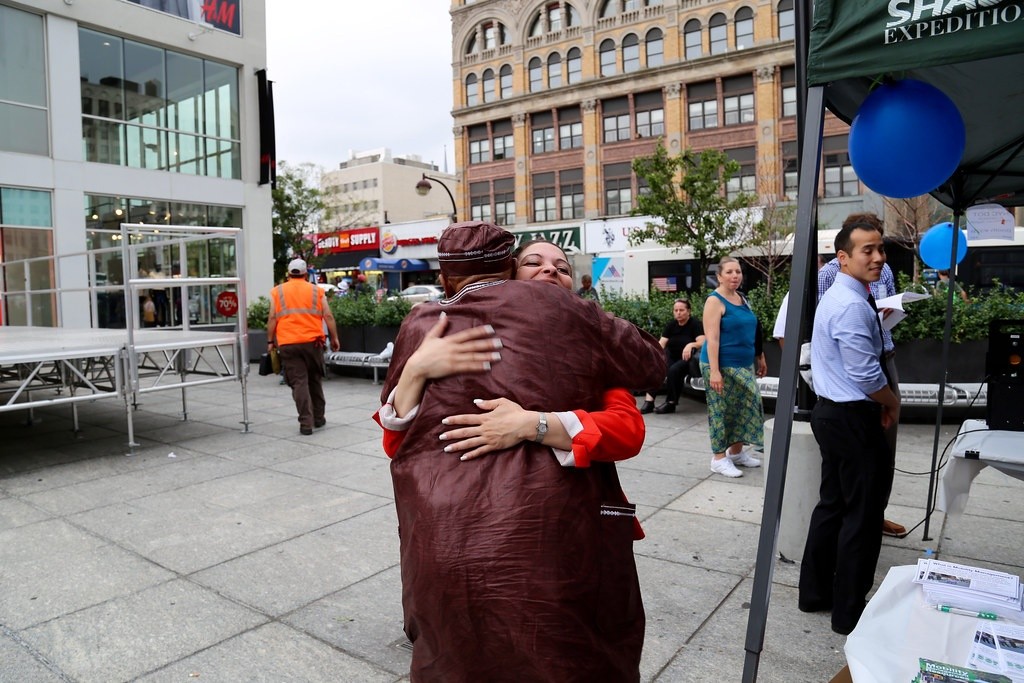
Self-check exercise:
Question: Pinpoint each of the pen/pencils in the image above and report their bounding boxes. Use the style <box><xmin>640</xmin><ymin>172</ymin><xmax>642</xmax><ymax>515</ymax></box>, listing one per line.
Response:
<box><xmin>932</xmin><ymin>603</ymin><xmax>1005</xmax><ymax>621</ymax></box>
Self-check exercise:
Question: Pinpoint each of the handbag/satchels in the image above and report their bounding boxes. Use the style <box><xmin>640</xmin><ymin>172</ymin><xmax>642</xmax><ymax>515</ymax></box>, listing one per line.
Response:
<box><xmin>271</xmin><ymin>351</ymin><xmax>280</xmax><ymax>374</ymax></box>
<box><xmin>258</xmin><ymin>353</ymin><xmax>273</xmax><ymax>375</ymax></box>
<box><xmin>738</xmin><ymin>292</ymin><xmax>763</xmax><ymax>357</ymax></box>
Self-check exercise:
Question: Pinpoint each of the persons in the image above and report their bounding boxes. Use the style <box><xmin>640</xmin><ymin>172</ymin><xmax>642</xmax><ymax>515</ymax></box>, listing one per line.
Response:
<box><xmin>818</xmin><ymin>212</ymin><xmax>909</xmax><ymax>538</ymax></box>
<box><xmin>377</xmin><ymin>274</ymin><xmax>384</xmax><ymax>288</ymax></box>
<box><xmin>138</xmin><ymin>261</ymin><xmax>148</xmax><ymax>328</ymax></box>
<box><xmin>771</xmin><ymin>254</ymin><xmax>825</xmax><ymax>412</ymax></box>
<box><xmin>937</xmin><ymin>269</ymin><xmax>968</xmax><ymax>300</ymax></box>
<box><xmin>698</xmin><ymin>255</ymin><xmax>766</xmax><ymax>478</ymax></box>
<box><xmin>379</xmin><ymin>219</ymin><xmax>671</xmax><ymax>683</ymax></box>
<box><xmin>637</xmin><ymin>298</ymin><xmax>705</xmax><ymax>414</ymax></box>
<box><xmin>797</xmin><ymin>223</ymin><xmax>904</xmax><ymax>634</ymax></box>
<box><xmin>370</xmin><ymin>240</ymin><xmax>645</xmax><ymax>543</ymax></box>
<box><xmin>354</xmin><ymin>274</ymin><xmax>375</xmax><ymax>301</ymax></box>
<box><xmin>266</xmin><ymin>259</ymin><xmax>340</xmax><ymax>435</ymax></box>
<box><xmin>576</xmin><ymin>274</ymin><xmax>600</xmax><ymax>301</ymax></box>
<box><xmin>148</xmin><ymin>263</ymin><xmax>171</xmax><ymax>328</ymax></box>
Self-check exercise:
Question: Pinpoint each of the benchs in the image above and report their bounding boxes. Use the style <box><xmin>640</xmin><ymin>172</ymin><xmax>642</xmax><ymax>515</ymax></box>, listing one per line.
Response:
<box><xmin>323</xmin><ymin>352</ymin><xmax>392</xmax><ymax>386</ymax></box>
<box><xmin>664</xmin><ymin>376</ymin><xmax>989</xmax><ymax>424</ymax></box>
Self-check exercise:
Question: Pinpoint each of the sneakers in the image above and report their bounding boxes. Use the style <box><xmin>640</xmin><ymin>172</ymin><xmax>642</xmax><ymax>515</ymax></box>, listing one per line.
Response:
<box><xmin>711</xmin><ymin>456</ymin><xmax>743</xmax><ymax>477</ymax></box>
<box><xmin>728</xmin><ymin>452</ymin><xmax>761</xmax><ymax>467</ymax></box>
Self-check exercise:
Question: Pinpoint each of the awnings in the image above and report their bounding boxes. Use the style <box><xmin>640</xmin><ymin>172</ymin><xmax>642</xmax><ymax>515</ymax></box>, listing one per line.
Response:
<box><xmin>358</xmin><ymin>257</ymin><xmax>396</xmax><ymax>292</ymax></box>
<box><xmin>394</xmin><ymin>258</ymin><xmax>428</xmax><ymax>292</ymax></box>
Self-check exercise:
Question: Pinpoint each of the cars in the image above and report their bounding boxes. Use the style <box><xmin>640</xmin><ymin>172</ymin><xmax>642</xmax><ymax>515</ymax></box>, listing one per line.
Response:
<box><xmin>387</xmin><ymin>285</ymin><xmax>446</xmax><ymax>304</ymax></box>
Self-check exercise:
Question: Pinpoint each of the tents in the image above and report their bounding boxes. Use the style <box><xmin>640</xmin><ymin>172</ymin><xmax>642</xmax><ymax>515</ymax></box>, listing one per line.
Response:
<box><xmin>741</xmin><ymin>2</ymin><xmax>1024</xmax><ymax>683</ymax></box>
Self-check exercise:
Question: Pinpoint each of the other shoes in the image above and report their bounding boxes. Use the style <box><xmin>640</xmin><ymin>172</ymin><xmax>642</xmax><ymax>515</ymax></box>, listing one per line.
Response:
<box><xmin>882</xmin><ymin>519</ymin><xmax>906</xmax><ymax>537</ymax></box>
<box><xmin>639</xmin><ymin>400</ymin><xmax>656</xmax><ymax>414</ymax></box>
<box><xmin>300</xmin><ymin>428</ymin><xmax>312</xmax><ymax>435</ymax></box>
<box><xmin>315</xmin><ymin>417</ymin><xmax>326</xmax><ymax>427</ymax></box>
<box><xmin>832</xmin><ymin>608</ymin><xmax>863</xmax><ymax>635</ymax></box>
<box><xmin>798</xmin><ymin>596</ymin><xmax>831</xmax><ymax>611</ymax></box>
<box><xmin>653</xmin><ymin>402</ymin><xmax>676</xmax><ymax>413</ymax></box>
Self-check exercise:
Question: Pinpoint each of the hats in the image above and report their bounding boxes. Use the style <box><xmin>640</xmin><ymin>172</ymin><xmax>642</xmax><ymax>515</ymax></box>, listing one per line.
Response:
<box><xmin>288</xmin><ymin>259</ymin><xmax>307</xmax><ymax>275</ymax></box>
<box><xmin>437</xmin><ymin>221</ymin><xmax>516</xmax><ymax>276</ymax></box>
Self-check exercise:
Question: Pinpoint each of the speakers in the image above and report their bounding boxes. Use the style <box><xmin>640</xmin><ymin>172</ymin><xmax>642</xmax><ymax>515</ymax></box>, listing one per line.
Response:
<box><xmin>986</xmin><ymin>319</ymin><xmax>1024</xmax><ymax>431</ymax></box>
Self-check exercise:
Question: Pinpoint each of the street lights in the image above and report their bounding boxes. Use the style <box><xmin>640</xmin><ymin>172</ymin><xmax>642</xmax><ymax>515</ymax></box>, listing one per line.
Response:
<box><xmin>414</xmin><ymin>172</ymin><xmax>457</xmax><ymax>224</ymax></box>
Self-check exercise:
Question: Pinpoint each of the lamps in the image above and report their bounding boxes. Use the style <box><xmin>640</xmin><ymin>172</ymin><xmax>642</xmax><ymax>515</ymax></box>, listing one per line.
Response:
<box><xmin>188</xmin><ymin>21</ymin><xmax>213</xmax><ymax>42</ymax></box>
<box><xmin>416</xmin><ymin>173</ymin><xmax>460</xmax><ymax>214</ymax></box>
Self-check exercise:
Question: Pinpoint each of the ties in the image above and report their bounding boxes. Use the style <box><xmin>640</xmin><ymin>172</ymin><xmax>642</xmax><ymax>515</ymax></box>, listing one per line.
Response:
<box><xmin>868</xmin><ymin>295</ymin><xmax>893</xmax><ymax>390</ymax></box>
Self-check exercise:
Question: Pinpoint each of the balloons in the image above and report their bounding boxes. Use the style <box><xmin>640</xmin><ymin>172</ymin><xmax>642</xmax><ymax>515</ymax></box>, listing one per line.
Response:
<box><xmin>918</xmin><ymin>222</ymin><xmax>968</xmax><ymax>271</ymax></box>
<box><xmin>848</xmin><ymin>79</ymin><xmax>965</xmax><ymax>199</ymax></box>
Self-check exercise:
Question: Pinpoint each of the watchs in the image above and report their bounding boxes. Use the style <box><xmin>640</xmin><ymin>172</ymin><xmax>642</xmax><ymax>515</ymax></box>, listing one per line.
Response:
<box><xmin>536</xmin><ymin>410</ymin><xmax>550</xmax><ymax>444</ymax></box>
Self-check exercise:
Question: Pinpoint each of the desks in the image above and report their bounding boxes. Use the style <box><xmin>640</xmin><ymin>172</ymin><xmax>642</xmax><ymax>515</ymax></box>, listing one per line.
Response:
<box><xmin>829</xmin><ymin>564</ymin><xmax>1024</xmax><ymax>683</ymax></box>
<box><xmin>934</xmin><ymin>419</ymin><xmax>1024</xmax><ymax>561</ymax></box>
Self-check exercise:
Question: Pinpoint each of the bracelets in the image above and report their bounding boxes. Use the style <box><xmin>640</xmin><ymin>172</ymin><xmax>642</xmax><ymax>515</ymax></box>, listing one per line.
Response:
<box><xmin>267</xmin><ymin>341</ymin><xmax>274</xmax><ymax>344</ymax></box>
